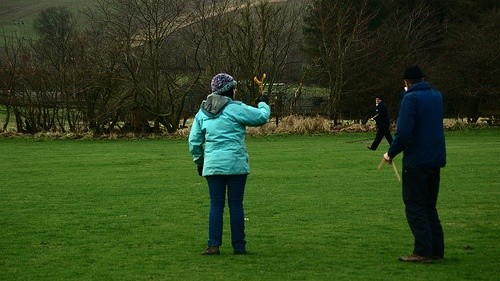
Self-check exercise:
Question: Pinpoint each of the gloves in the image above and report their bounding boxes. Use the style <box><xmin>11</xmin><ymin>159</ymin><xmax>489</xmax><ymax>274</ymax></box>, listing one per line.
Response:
<box><xmin>193</xmin><ymin>156</ymin><xmax>203</xmax><ymax>176</ymax></box>
<box><xmin>258</xmin><ymin>95</ymin><xmax>268</xmax><ymax>104</ymax></box>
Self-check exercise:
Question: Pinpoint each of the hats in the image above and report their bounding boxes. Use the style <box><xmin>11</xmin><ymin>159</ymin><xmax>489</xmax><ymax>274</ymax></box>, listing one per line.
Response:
<box><xmin>210</xmin><ymin>73</ymin><xmax>238</xmax><ymax>94</ymax></box>
<box><xmin>375</xmin><ymin>95</ymin><xmax>384</xmax><ymax>101</ymax></box>
<box><xmin>399</xmin><ymin>66</ymin><xmax>425</xmax><ymax>79</ymax></box>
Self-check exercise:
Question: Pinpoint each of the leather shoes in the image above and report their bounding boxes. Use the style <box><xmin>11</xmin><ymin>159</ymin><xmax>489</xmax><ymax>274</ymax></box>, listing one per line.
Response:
<box><xmin>398</xmin><ymin>254</ymin><xmax>437</xmax><ymax>262</ymax></box>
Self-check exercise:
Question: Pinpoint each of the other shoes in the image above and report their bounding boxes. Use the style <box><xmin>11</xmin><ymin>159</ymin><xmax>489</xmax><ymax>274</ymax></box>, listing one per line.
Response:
<box><xmin>201</xmin><ymin>246</ymin><xmax>221</xmax><ymax>255</ymax></box>
<box><xmin>389</xmin><ymin>143</ymin><xmax>394</xmax><ymax>150</ymax></box>
<box><xmin>367</xmin><ymin>146</ymin><xmax>375</xmax><ymax>150</ymax></box>
<box><xmin>233</xmin><ymin>248</ymin><xmax>248</xmax><ymax>254</ymax></box>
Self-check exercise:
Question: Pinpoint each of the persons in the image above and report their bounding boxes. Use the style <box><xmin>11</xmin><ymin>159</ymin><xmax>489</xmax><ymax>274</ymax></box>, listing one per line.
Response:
<box><xmin>188</xmin><ymin>74</ymin><xmax>270</xmax><ymax>255</ymax></box>
<box><xmin>367</xmin><ymin>96</ymin><xmax>393</xmax><ymax>151</ymax></box>
<box><xmin>384</xmin><ymin>66</ymin><xmax>447</xmax><ymax>263</ymax></box>
